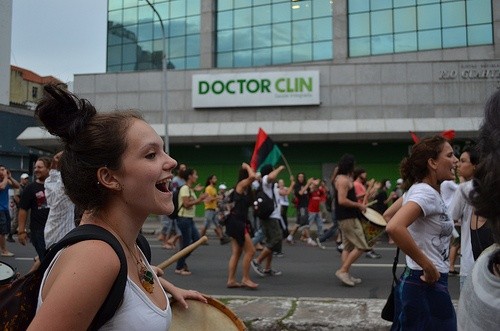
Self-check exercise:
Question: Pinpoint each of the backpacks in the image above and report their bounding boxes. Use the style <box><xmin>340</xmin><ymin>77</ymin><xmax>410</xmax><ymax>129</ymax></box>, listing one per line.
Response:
<box><xmin>0</xmin><ymin>225</ymin><xmax>127</xmax><ymax>331</ymax></box>
<box><xmin>252</xmin><ymin>180</ymin><xmax>278</xmax><ymax>220</ymax></box>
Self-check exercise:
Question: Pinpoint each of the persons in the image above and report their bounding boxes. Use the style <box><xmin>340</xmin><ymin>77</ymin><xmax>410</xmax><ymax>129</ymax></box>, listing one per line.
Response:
<box><xmin>0</xmin><ymin>82</ymin><xmax>500</xmax><ymax>331</ymax></box>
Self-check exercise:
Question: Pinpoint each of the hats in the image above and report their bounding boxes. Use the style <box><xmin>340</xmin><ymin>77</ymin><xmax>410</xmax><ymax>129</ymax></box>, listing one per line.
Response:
<box><xmin>261</xmin><ymin>164</ymin><xmax>273</xmax><ymax>171</ymax></box>
<box><xmin>21</xmin><ymin>174</ymin><xmax>29</xmax><ymax>179</ymax></box>
<box><xmin>219</xmin><ymin>184</ymin><xmax>227</xmax><ymax>190</ymax></box>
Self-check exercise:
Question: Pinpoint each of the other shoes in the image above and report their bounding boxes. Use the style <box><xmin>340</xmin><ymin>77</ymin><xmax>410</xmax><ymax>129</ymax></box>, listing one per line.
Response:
<box><xmin>220</xmin><ymin>235</ymin><xmax>231</xmax><ymax>244</ymax></box>
<box><xmin>242</xmin><ymin>279</ymin><xmax>258</xmax><ymax>288</ymax></box>
<box><xmin>227</xmin><ymin>282</ymin><xmax>242</xmax><ymax>287</ymax></box>
<box><xmin>263</xmin><ymin>269</ymin><xmax>282</xmax><ymax>275</ymax></box>
<box><xmin>7</xmin><ymin>237</ymin><xmax>16</xmax><ymax>243</ymax></box>
<box><xmin>251</xmin><ymin>260</ymin><xmax>264</xmax><ymax>276</ymax></box>
<box><xmin>273</xmin><ymin>252</ymin><xmax>285</xmax><ymax>257</ymax></box>
<box><xmin>350</xmin><ymin>275</ymin><xmax>362</xmax><ymax>284</ymax></box>
<box><xmin>336</xmin><ymin>269</ymin><xmax>355</xmax><ymax>286</ymax></box>
<box><xmin>160</xmin><ymin>234</ymin><xmax>166</xmax><ymax>239</ymax></box>
<box><xmin>448</xmin><ymin>271</ymin><xmax>460</xmax><ymax>276</ymax></box>
<box><xmin>175</xmin><ymin>268</ymin><xmax>191</xmax><ymax>274</ymax></box>
<box><xmin>162</xmin><ymin>243</ymin><xmax>175</xmax><ymax>248</ymax></box>
<box><xmin>287</xmin><ymin>235</ymin><xmax>380</xmax><ymax>259</ymax></box>
<box><xmin>1</xmin><ymin>251</ymin><xmax>14</xmax><ymax>256</ymax></box>
<box><xmin>255</xmin><ymin>242</ymin><xmax>264</xmax><ymax>250</ymax></box>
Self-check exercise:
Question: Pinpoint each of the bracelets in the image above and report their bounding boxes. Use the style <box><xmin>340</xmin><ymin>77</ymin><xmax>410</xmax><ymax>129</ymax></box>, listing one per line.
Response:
<box><xmin>18</xmin><ymin>231</ymin><xmax>26</xmax><ymax>235</ymax></box>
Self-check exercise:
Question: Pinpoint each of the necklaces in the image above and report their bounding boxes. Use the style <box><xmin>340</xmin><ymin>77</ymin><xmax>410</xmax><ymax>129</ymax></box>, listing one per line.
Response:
<box><xmin>108</xmin><ymin>225</ymin><xmax>155</xmax><ymax>294</ymax></box>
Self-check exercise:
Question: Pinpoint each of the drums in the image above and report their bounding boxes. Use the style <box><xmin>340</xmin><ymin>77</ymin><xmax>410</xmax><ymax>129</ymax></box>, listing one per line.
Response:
<box><xmin>342</xmin><ymin>207</ymin><xmax>388</xmax><ymax>253</ymax></box>
<box><xmin>0</xmin><ymin>260</ymin><xmax>18</xmax><ymax>296</ymax></box>
<box><xmin>166</xmin><ymin>294</ymin><xmax>249</xmax><ymax>331</ymax></box>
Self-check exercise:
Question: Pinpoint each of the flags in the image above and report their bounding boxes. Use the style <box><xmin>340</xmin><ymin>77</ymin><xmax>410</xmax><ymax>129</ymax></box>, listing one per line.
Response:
<box><xmin>250</xmin><ymin>128</ymin><xmax>281</xmax><ymax>174</ymax></box>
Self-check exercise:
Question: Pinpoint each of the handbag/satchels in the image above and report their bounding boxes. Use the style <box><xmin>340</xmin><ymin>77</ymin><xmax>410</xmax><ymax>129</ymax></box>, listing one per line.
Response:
<box><xmin>381</xmin><ymin>288</ymin><xmax>395</xmax><ymax>322</ymax></box>
<box><xmin>169</xmin><ymin>209</ymin><xmax>178</xmax><ymax>219</ymax></box>
